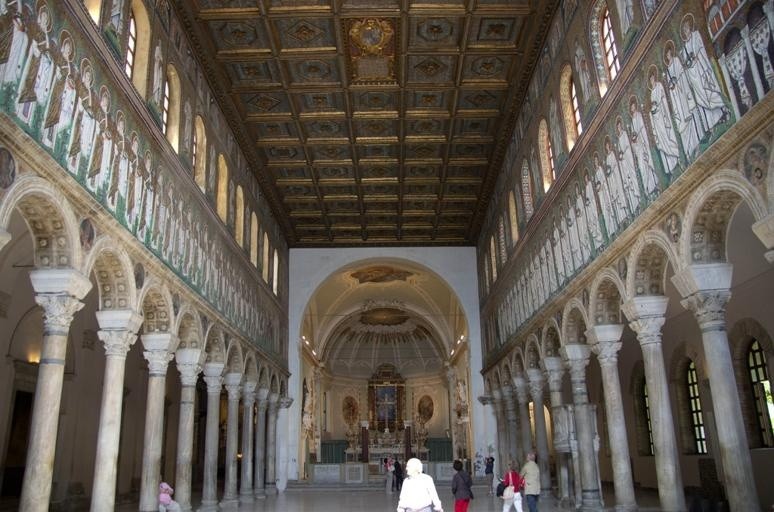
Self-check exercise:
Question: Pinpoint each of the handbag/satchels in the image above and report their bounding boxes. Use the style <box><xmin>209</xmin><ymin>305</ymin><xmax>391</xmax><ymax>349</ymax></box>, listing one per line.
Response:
<box><xmin>499</xmin><ymin>472</ymin><xmax>515</xmax><ymax>500</ymax></box>
<box><xmin>468</xmin><ymin>489</ymin><xmax>476</xmax><ymax>500</ymax></box>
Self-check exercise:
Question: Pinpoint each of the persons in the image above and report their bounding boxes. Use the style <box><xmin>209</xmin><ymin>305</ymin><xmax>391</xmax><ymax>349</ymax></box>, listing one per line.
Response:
<box><xmin>159</xmin><ymin>474</ymin><xmax>180</xmax><ymax>512</ymax></box>
<box><xmin>384</xmin><ymin>453</ymin><xmax>541</xmax><ymax>512</ymax></box>
